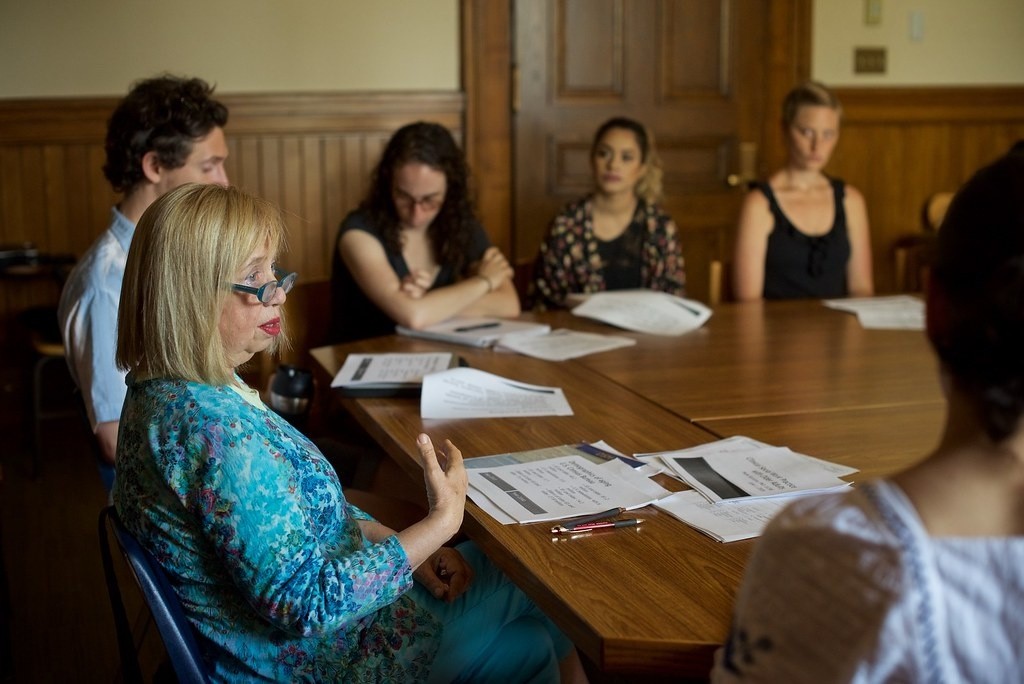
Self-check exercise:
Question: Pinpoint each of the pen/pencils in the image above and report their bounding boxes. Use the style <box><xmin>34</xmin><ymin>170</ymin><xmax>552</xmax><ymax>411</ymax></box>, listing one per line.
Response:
<box><xmin>548</xmin><ymin>506</ymin><xmax>647</xmax><ymax>534</ymax></box>
<box><xmin>455</xmin><ymin>322</ymin><xmax>501</xmax><ymax>332</ymax></box>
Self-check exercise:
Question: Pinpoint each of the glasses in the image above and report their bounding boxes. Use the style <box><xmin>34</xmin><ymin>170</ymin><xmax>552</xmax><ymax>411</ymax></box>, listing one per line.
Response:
<box><xmin>220</xmin><ymin>262</ymin><xmax>298</xmax><ymax>303</ymax></box>
<box><xmin>389</xmin><ymin>183</ymin><xmax>445</xmax><ymax>211</ymax></box>
<box><xmin>790</xmin><ymin>122</ymin><xmax>834</xmax><ymax>143</ymax></box>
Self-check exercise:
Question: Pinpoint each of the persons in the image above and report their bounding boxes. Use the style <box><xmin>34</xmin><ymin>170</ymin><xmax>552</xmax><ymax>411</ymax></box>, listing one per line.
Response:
<box><xmin>114</xmin><ymin>183</ymin><xmax>589</xmax><ymax>684</ymax></box>
<box><xmin>59</xmin><ymin>78</ymin><xmax>468</xmax><ymax>547</ymax></box>
<box><xmin>711</xmin><ymin>142</ymin><xmax>1023</xmax><ymax>684</ymax></box>
<box><xmin>732</xmin><ymin>82</ymin><xmax>874</xmax><ymax>300</ymax></box>
<box><xmin>329</xmin><ymin>121</ymin><xmax>521</xmax><ymax>344</ymax></box>
<box><xmin>533</xmin><ymin>116</ymin><xmax>687</xmax><ymax>311</ymax></box>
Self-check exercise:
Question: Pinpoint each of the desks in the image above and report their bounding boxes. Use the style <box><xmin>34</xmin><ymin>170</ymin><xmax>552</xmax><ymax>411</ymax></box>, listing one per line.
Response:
<box><xmin>309</xmin><ymin>294</ymin><xmax>948</xmax><ymax>681</ymax></box>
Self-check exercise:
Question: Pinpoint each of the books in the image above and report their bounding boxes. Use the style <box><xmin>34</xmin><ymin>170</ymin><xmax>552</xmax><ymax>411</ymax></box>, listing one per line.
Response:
<box><xmin>395</xmin><ymin>317</ymin><xmax>552</xmax><ymax>347</ymax></box>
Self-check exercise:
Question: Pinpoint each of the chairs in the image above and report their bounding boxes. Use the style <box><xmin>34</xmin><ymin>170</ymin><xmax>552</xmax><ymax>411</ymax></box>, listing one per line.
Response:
<box><xmin>99</xmin><ymin>510</ymin><xmax>209</xmax><ymax>684</ymax></box>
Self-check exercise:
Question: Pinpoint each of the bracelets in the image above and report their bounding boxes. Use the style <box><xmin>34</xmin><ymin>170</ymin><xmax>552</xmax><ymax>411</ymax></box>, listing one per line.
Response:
<box><xmin>473</xmin><ymin>273</ymin><xmax>492</xmax><ymax>292</ymax></box>
